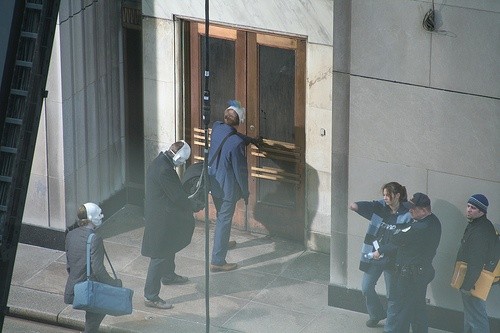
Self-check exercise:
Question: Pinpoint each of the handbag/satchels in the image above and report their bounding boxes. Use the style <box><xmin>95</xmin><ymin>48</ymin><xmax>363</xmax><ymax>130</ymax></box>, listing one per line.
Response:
<box><xmin>181</xmin><ymin>160</ymin><xmax>209</xmax><ymax>211</ymax></box>
<box><xmin>73</xmin><ymin>281</ymin><xmax>133</xmax><ymax>315</ymax></box>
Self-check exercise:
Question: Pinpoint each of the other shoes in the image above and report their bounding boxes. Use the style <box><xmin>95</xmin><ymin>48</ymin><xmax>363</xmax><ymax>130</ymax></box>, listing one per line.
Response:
<box><xmin>228</xmin><ymin>241</ymin><xmax>235</xmax><ymax>248</ymax></box>
<box><xmin>367</xmin><ymin>314</ymin><xmax>386</xmax><ymax>326</ymax></box>
<box><xmin>210</xmin><ymin>263</ymin><xmax>236</xmax><ymax>272</ymax></box>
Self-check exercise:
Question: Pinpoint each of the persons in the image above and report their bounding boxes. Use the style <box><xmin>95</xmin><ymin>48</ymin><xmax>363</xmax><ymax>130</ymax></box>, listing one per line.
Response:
<box><xmin>140</xmin><ymin>139</ymin><xmax>193</xmax><ymax>309</ymax></box>
<box><xmin>65</xmin><ymin>202</ymin><xmax>122</xmax><ymax>333</ymax></box>
<box><xmin>454</xmin><ymin>194</ymin><xmax>500</xmax><ymax>333</ymax></box>
<box><xmin>350</xmin><ymin>182</ymin><xmax>416</xmax><ymax>328</ymax></box>
<box><xmin>373</xmin><ymin>192</ymin><xmax>441</xmax><ymax>333</ymax></box>
<box><xmin>208</xmin><ymin>106</ymin><xmax>264</xmax><ymax>273</ymax></box>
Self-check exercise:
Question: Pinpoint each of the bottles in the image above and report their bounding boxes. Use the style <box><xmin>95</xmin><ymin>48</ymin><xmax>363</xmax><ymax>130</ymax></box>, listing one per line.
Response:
<box><xmin>364</xmin><ymin>252</ymin><xmax>384</xmax><ymax>259</ymax></box>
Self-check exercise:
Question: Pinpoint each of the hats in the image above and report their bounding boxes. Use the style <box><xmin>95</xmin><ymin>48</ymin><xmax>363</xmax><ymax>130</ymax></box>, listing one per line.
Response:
<box><xmin>467</xmin><ymin>194</ymin><xmax>489</xmax><ymax>214</ymax></box>
<box><xmin>402</xmin><ymin>192</ymin><xmax>430</xmax><ymax>209</ymax></box>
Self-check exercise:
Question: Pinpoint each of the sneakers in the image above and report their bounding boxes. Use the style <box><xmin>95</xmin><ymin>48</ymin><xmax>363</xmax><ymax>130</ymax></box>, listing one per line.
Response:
<box><xmin>145</xmin><ymin>297</ymin><xmax>172</xmax><ymax>309</ymax></box>
<box><xmin>162</xmin><ymin>275</ymin><xmax>189</xmax><ymax>285</ymax></box>
